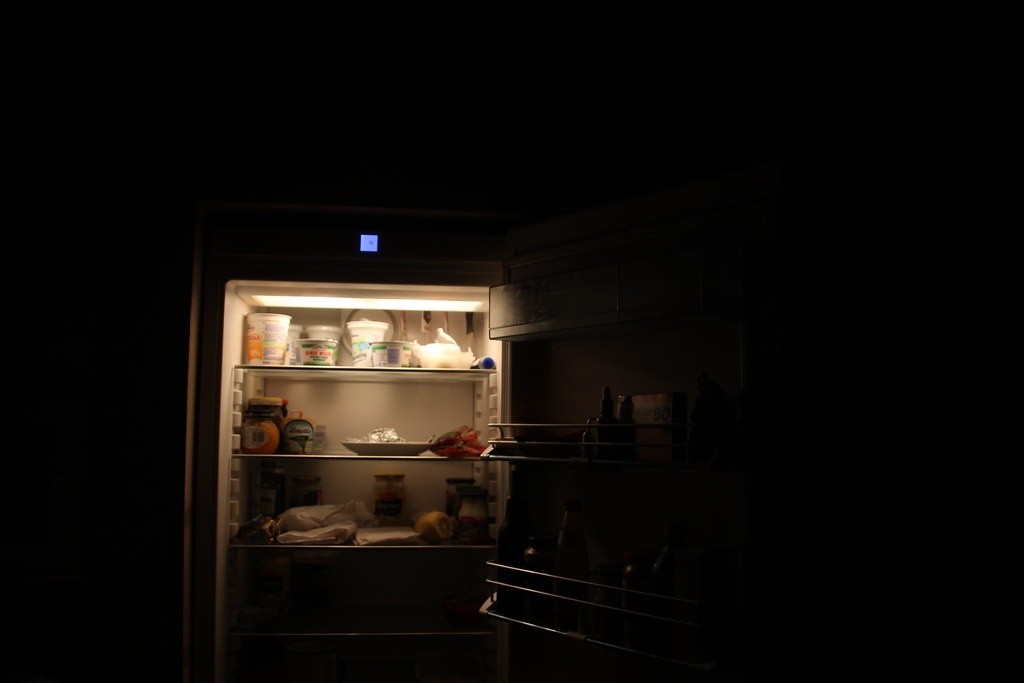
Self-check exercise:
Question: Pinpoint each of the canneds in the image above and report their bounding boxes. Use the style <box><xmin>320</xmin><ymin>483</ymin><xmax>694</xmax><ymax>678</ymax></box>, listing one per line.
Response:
<box><xmin>445</xmin><ymin>478</ymin><xmax>490</xmax><ymax>543</ymax></box>
<box><xmin>253</xmin><ymin>468</ymin><xmax>286</xmax><ymax>516</ymax></box>
<box><xmin>295</xmin><ymin>476</ymin><xmax>321</xmax><ymax>506</ymax></box>
<box><xmin>374</xmin><ymin>474</ymin><xmax>405</xmax><ymax>523</ymax></box>
<box><xmin>241</xmin><ymin>412</ymin><xmax>279</xmax><ymax>454</ymax></box>
<box><xmin>250</xmin><ymin>397</ymin><xmax>283</xmax><ymax>451</ymax></box>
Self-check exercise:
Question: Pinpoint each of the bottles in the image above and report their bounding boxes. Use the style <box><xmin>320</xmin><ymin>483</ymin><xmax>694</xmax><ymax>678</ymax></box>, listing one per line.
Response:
<box><xmin>595</xmin><ymin>376</ymin><xmax>637</xmax><ymax>458</ymax></box>
<box><xmin>497</xmin><ymin>461</ymin><xmax>688</xmax><ymax>657</ymax></box>
<box><xmin>445</xmin><ymin>474</ymin><xmax>490</xmax><ymax>544</ymax></box>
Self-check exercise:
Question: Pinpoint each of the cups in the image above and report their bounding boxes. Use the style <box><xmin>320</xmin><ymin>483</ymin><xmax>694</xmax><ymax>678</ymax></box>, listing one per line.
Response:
<box><xmin>246</xmin><ymin>311</ymin><xmax>414</xmax><ymax>369</ymax></box>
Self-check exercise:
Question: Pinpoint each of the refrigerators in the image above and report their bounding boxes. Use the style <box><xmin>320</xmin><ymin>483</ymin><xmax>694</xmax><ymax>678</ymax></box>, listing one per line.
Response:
<box><xmin>175</xmin><ymin>163</ymin><xmax>769</xmax><ymax>683</ymax></box>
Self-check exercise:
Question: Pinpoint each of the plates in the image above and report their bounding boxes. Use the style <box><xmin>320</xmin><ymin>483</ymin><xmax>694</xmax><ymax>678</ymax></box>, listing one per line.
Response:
<box><xmin>338</xmin><ymin>438</ymin><xmax>436</xmax><ymax>455</ymax></box>
